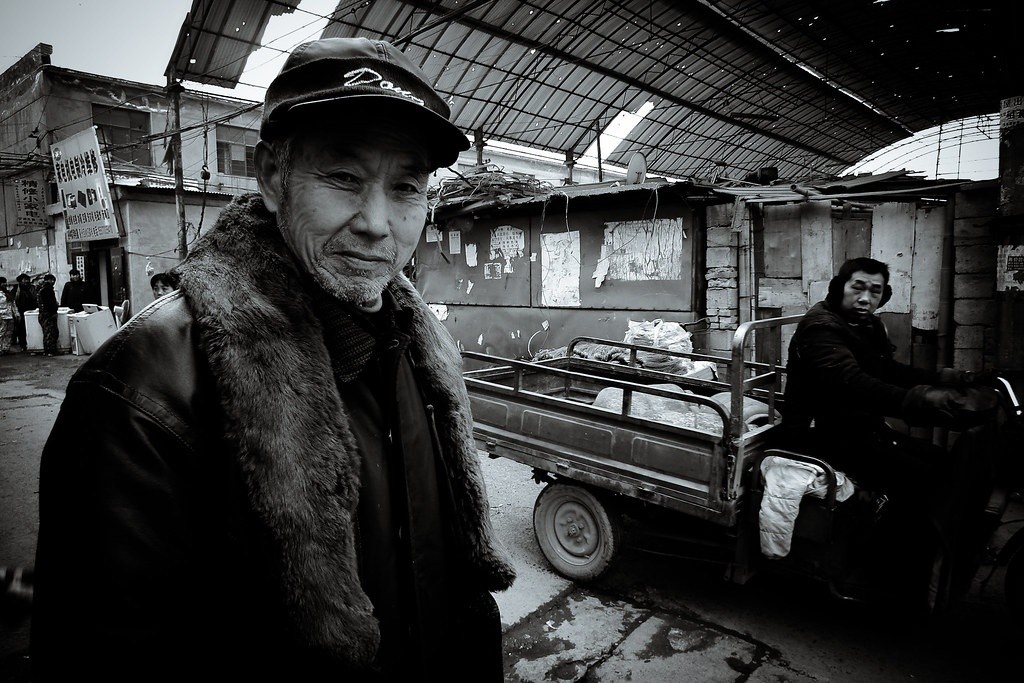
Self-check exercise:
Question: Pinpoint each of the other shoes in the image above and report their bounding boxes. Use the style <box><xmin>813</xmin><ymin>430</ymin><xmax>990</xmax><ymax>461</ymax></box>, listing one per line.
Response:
<box><xmin>44</xmin><ymin>351</ymin><xmax>64</xmax><ymax>357</ymax></box>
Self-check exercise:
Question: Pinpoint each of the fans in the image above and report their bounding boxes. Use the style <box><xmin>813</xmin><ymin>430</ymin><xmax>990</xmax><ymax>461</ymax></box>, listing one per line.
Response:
<box><xmin>113</xmin><ymin>300</ymin><xmax>129</xmax><ymax>330</ymax></box>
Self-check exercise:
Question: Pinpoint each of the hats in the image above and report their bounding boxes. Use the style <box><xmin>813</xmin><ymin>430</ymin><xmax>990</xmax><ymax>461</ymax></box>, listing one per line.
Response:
<box><xmin>260</xmin><ymin>35</ymin><xmax>469</xmax><ymax>166</ymax></box>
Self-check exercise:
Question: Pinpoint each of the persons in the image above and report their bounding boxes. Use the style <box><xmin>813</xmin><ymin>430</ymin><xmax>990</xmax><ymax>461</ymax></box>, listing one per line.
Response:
<box><xmin>27</xmin><ymin>37</ymin><xmax>513</xmax><ymax>683</ymax></box>
<box><xmin>150</xmin><ymin>273</ymin><xmax>175</xmax><ymax>299</ymax></box>
<box><xmin>60</xmin><ymin>269</ymin><xmax>89</xmax><ymax>313</ymax></box>
<box><xmin>0</xmin><ymin>274</ymin><xmax>36</xmax><ymax>355</ymax></box>
<box><xmin>781</xmin><ymin>258</ymin><xmax>978</xmax><ymax>606</ymax></box>
<box><xmin>36</xmin><ymin>274</ymin><xmax>65</xmax><ymax>356</ymax></box>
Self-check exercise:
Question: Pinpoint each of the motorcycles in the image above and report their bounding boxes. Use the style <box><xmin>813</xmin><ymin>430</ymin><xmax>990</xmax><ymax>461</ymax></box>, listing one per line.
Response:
<box><xmin>458</xmin><ymin>313</ymin><xmax>1023</xmax><ymax>617</ymax></box>
